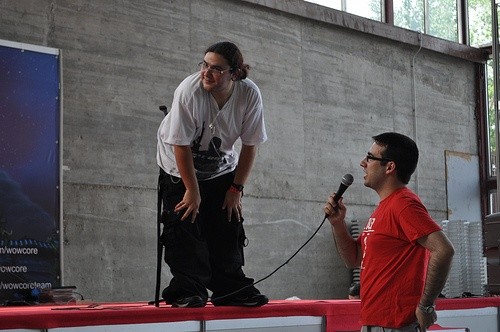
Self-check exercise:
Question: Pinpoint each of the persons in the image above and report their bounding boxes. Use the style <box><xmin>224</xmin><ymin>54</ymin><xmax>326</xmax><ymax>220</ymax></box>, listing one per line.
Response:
<box><xmin>324</xmin><ymin>132</ymin><xmax>455</xmax><ymax>332</ymax></box>
<box><xmin>156</xmin><ymin>41</ymin><xmax>268</xmax><ymax>308</ymax></box>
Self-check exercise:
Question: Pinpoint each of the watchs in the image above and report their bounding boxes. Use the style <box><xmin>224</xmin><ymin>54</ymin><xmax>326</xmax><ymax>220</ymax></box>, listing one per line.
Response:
<box><xmin>419</xmin><ymin>304</ymin><xmax>435</xmax><ymax>313</ymax></box>
<box><xmin>229</xmin><ymin>183</ymin><xmax>244</xmax><ymax>192</ymax></box>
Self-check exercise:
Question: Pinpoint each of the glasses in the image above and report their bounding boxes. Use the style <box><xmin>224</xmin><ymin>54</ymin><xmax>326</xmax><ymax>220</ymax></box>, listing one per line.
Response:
<box><xmin>198</xmin><ymin>62</ymin><xmax>233</xmax><ymax>74</ymax></box>
<box><xmin>366</xmin><ymin>153</ymin><xmax>384</xmax><ymax>162</ymax></box>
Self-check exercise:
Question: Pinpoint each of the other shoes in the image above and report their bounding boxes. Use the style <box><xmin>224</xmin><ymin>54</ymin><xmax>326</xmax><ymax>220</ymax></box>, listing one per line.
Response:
<box><xmin>172</xmin><ymin>297</ymin><xmax>206</xmax><ymax>308</ymax></box>
<box><xmin>212</xmin><ymin>294</ymin><xmax>268</xmax><ymax>307</ymax></box>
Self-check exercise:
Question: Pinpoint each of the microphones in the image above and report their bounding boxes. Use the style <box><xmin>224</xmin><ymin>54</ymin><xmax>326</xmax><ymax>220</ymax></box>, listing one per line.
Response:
<box><xmin>325</xmin><ymin>174</ymin><xmax>354</xmax><ymax>217</ymax></box>
